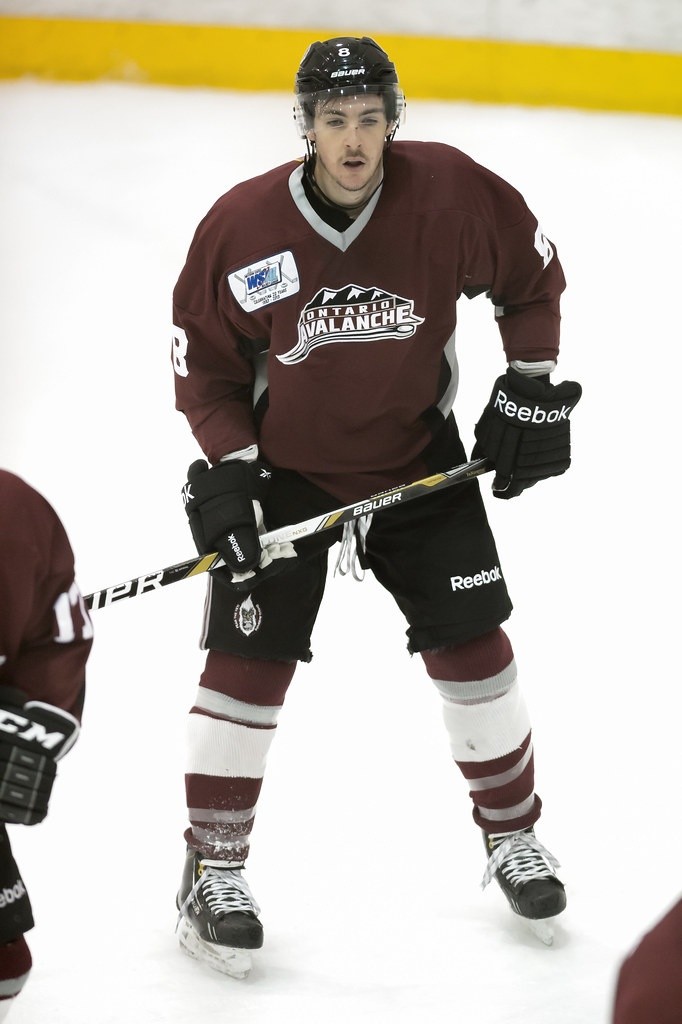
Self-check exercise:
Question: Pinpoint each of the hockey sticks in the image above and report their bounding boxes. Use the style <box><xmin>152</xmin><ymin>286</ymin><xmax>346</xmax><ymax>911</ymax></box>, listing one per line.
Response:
<box><xmin>77</xmin><ymin>456</ymin><xmax>491</xmax><ymax>609</ymax></box>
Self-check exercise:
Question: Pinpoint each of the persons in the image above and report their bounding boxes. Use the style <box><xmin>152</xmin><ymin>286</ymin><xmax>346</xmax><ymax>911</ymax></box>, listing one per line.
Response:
<box><xmin>0</xmin><ymin>466</ymin><xmax>95</xmax><ymax>1024</ymax></box>
<box><xmin>171</xmin><ymin>32</ymin><xmax>582</xmax><ymax>979</ymax></box>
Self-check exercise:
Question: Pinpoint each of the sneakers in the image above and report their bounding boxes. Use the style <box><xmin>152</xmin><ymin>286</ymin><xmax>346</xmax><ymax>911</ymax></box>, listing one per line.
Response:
<box><xmin>176</xmin><ymin>828</ymin><xmax>263</xmax><ymax>979</ymax></box>
<box><xmin>472</xmin><ymin>794</ymin><xmax>566</xmax><ymax>946</ymax></box>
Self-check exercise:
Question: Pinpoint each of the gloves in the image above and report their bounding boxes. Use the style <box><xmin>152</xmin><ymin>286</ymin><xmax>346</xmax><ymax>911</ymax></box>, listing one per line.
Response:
<box><xmin>0</xmin><ymin>700</ymin><xmax>81</xmax><ymax>826</ymax></box>
<box><xmin>180</xmin><ymin>460</ymin><xmax>298</xmax><ymax>592</ymax></box>
<box><xmin>470</xmin><ymin>367</ymin><xmax>582</xmax><ymax>500</ymax></box>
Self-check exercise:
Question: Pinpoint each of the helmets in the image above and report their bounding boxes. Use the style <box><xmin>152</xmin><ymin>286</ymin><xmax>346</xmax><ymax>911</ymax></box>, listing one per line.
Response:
<box><xmin>296</xmin><ymin>36</ymin><xmax>399</xmax><ymax>125</ymax></box>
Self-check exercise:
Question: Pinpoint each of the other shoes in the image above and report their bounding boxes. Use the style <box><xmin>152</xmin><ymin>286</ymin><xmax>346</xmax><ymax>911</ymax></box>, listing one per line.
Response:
<box><xmin>0</xmin><ymin>935</ymin><xmax>32</xmax><ymax>1000</ymax></box>
